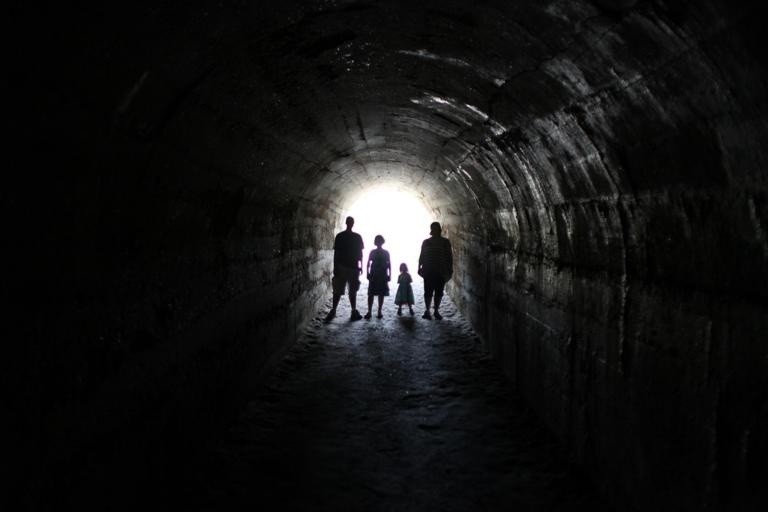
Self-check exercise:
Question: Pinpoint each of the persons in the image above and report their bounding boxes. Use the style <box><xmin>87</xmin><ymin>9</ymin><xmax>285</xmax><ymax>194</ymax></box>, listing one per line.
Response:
<box><xmin>417</xmin><ymin>222</ymin><xmax>453</xmax><ymax>320</ymax></box>
<box><xmin>365</xmin><ymin>235</ymin><xmax>391</xmax><ymax>319</ymax></box>
<box><xmin>394</xmin><ymin>263</ymin><xmax>415</xmax><ymax>315</ymax></box>
<box><xmin>324</xmin><ymin>216</ymin><xmax>364</xmax><ymax>322</ymax></box>
